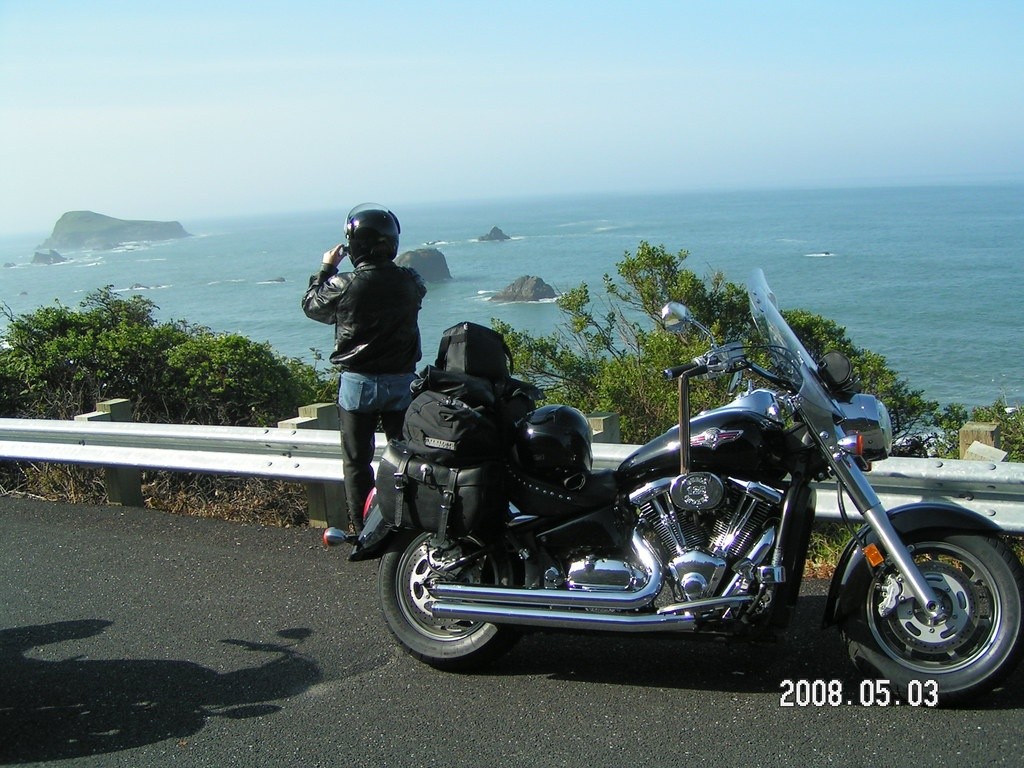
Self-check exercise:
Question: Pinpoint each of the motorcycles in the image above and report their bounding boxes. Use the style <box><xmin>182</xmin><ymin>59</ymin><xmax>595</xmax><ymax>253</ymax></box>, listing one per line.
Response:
<box><xmin>321</xmin><ymin>267</ymin><xmax>1023</xmax><ymax>709</ymax></box>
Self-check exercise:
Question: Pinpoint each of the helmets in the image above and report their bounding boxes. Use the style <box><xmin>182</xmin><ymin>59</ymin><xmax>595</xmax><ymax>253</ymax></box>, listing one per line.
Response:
<box><xmin>513</xmin><ymin>402</ymin><xmax>593</xmax><ymax>484</ymax></box>
<box><xmin>344</xmin><ymin>203</ymin><xmax>400</xmax><ymax>267</ymax></box>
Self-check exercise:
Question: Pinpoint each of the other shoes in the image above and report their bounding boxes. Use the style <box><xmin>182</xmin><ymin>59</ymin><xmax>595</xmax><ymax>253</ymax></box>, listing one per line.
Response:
<box><xmin>342</xmin><ymin>521</ymin><xmax>362</xmax><ymax>545</ymax></box>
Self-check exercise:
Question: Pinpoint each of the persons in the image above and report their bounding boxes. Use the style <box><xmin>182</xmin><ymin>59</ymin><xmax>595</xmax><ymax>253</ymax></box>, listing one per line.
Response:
<box><xmin>302</xmin><ymin>201</ymin><xmax>427</xmax><ymax>542</ymax></box>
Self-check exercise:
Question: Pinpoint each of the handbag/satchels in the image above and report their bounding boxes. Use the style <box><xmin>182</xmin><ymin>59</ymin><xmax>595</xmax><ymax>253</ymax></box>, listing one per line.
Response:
<box><xmin>434</xmin><ymin>321</ymin><xmax>515</xmax><ymax>380</ymax></box>
<box><xmin>375</xmin><ymin>439</ymin><xmax>487</xmax><ymax>545</ymax></box>
<box><xmin>401</xmin><ymin>389</ymin><xmax>506</xmax><ymax>469</ymax></box>
